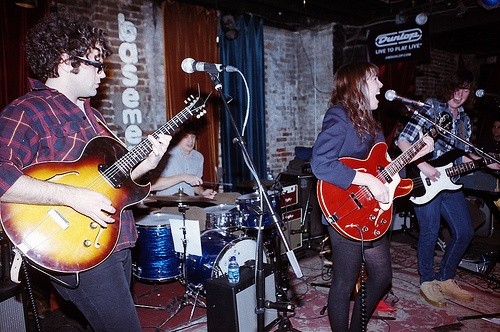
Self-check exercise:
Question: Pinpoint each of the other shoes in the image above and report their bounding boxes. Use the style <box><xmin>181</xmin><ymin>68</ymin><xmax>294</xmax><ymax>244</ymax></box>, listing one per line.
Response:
<box><xmin>419</xmin><ymin>280</ymin><xmax>448</xmax><ymax>308</ymax></box>
<box><xmin>433</xmin><ymin>278</ymin><xmax>475</xmax><ymax>303</ymax></box>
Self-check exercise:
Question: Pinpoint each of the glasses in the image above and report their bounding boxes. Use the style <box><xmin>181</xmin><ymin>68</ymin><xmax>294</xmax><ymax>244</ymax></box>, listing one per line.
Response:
<box><xmin>74</xmin><ymin>55</ymin><xmax>106</xmax><ymax>74</ymax></box>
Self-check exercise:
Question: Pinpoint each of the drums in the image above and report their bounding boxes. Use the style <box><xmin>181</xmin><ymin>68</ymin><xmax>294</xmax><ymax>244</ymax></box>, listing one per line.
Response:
<box><xmin>179</xmin><ymin>230</ymin><xmax>268</xmax><ymax>295</ymax></box>
<box><xmin>132</xmin><ymin>214</ymin><xmax>186</xmax><ymax>281</ymax></box>
<box><xmin>237</xmin><ymin>190</ymin><xmax>280</xmax><ymax>229</ymax></box>
<box><xmin>203</xmin><ymin>205</ymin><xmax>242</xmax><ymax>230</ymax></box>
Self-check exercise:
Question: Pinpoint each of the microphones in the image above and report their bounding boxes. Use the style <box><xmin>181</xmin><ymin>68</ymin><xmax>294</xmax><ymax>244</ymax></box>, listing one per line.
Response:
<box><xmin>385</xmin><ymin>89</ymin><xmax>434</xmax><ymax>109</ymax></box>
<box><xmin>290</xmin><ymin>229</ymin><xmax>310</xmax><ymax>235</ymax></box>
<box><xmin>476</xmin><ymin>89</ymin><xmax>500</xmax><ymax>98</ymax></box>
<box><xmin>181</xmin><ymin>58</ymin><xmax>238</xmax><ymax>73</ymax></box>
<box><xmin>264</xmin><ymin>300</ymin><xmax>295</xmax><ymax>311</ymax></box>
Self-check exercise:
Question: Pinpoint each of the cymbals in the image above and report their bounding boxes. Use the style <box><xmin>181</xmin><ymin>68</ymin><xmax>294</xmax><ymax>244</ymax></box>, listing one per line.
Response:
<box><xmin>146</xmin><ymin>193</ymin><xmax>217</xmax><ymax>202</ymax></box>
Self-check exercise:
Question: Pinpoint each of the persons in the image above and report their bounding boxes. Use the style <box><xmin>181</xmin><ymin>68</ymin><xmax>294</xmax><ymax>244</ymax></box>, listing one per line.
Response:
<box><xmin>147</xmin><ymin>124</ymin><xmax>217</xmax><ymax>200</ymax></box>
<box><xmin>309</xmin><ymin>61</ymin><xmax>434</xmax><ymax>332</ymax></box>
<box><xmin>394</xmin><ymin>68</ymin><xmax>500</xmax><ymax>308</ymax></box>
<box><xmin>0</xmin><ymin>9</ymin><xmax>172</xmax><ymax>332</ymax></box>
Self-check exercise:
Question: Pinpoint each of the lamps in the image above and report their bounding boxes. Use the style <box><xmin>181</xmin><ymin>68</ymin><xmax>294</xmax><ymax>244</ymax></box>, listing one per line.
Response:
<box><xmin>221</xmin><ymin>14</ymin><xmax>238</xmax><ymax>41</ymax></box>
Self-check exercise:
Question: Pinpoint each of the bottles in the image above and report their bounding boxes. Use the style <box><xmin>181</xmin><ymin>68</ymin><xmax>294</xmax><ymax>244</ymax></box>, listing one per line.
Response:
<box><xmin>228</xmin><ymin>256</ymin><xmax>240</xmax><ymax>284</ymax></box>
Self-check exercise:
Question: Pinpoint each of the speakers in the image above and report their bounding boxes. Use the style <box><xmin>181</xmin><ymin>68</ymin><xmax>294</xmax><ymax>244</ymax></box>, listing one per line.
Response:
<box><xmin>207</xmin><ymin>260</ymin><xmax>281</xmax><ymax>332</ymax></box>
<box><xmin>276</xmin><ymin>170</ymin><xmax>328</xmax><ymax>256</ymax></box>
<box><xmin>0</xmin><ymin>280</ymin><xmax>27</xmax><ymax>332</ymax></box>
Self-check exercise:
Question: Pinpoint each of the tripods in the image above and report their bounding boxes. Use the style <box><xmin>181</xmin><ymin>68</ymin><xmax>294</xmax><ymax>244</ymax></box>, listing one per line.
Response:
<box><xmin>157</xmin><ymin>204</ymin><xmax>207</xmax><ymax>332</ymax></box>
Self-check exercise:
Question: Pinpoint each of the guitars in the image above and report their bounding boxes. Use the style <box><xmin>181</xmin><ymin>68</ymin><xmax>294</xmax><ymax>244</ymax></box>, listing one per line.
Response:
<box><xmin>316</xmin><ymin>111</ymin><xmax>453</xmax><ymax>241</ymax></box>
<box><xmin>0</xmin><ymin>94</ymin><xmax>208</xmax><ymax>274</ymax></box>
<box><xmin>407</xmin><ymin>149</ymin><xmax>500</xmax><ymax>205</ymax></box>
